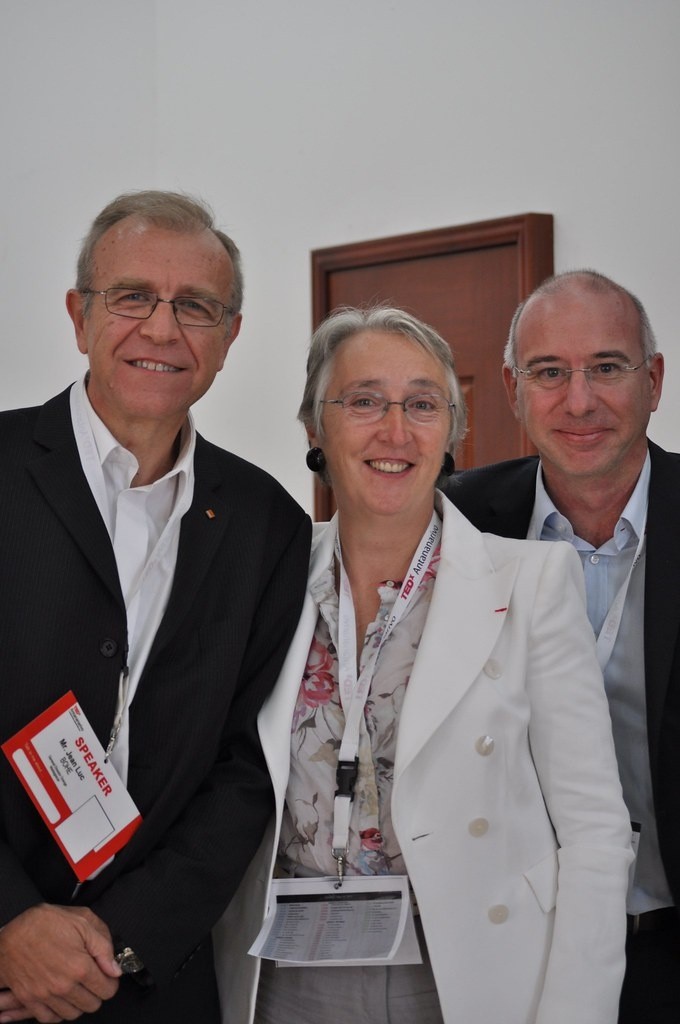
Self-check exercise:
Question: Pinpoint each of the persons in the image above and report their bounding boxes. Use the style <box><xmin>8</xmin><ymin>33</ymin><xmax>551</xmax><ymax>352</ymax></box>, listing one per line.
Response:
<box><xmin>210</xmin><ymin>308</ymin><xmax>632</xmax><ymax>1023</ymax></box>
<box><xmin>0</xmin><ymin>189</ymin><xmax>315</xmax><ymax>1023</ymax></box>
<box><xmin>435</xmin><ymin>269</ymin><xmax>680</xmax><ymax>1023</ymax></box>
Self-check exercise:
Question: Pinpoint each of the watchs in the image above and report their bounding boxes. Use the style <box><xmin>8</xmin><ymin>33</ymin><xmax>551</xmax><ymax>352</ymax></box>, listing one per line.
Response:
<box><xmin>112</xmin><ymin>937</ymin><xmax>145</xmax><ymax>974</ymax></box>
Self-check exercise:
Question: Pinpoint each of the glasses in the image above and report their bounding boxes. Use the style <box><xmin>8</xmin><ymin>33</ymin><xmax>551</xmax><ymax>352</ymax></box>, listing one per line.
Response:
<box><xmin>515</xmin><ymin>357</ymin><xmax>652</xmax><ymax>390</ymax></box>
<box><xmin>80</xmin><ymin>287</ymin><xmax>236</xmax><ymax>327</ymax></box>
<box><xmin>318</xmin><ymin>392</ymin><xmax>455</xmax><ymax>426</ymax></box>
